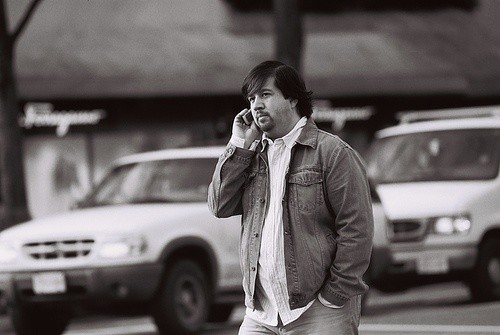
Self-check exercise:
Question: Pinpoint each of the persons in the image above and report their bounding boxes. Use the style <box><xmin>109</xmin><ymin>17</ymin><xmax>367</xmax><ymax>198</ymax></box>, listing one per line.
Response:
<box><xmin>206</xmin><ymin>58</ymin><xmax>375</xmax><ymax>335</ymax></box>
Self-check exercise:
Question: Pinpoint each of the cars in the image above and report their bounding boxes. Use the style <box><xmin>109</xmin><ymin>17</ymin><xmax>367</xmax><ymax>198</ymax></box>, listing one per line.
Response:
<box><xmin>0</xmin><ymin>141</ymin><xmax>396</xmax><ymax>334</ymax></box>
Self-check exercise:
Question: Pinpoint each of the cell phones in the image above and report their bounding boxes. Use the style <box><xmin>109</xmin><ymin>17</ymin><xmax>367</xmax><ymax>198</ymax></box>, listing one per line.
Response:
<box><xmin>242</xmin><ymin>108</ymin><xmax>254</xmax><ymax>125</ymax></box>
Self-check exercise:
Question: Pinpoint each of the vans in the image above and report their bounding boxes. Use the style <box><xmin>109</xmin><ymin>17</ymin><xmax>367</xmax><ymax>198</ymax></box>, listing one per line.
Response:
<box><xmin>364</xmin><ymin>116</ymin><xmax>499</xmax><ymax>301</ymax></box>
